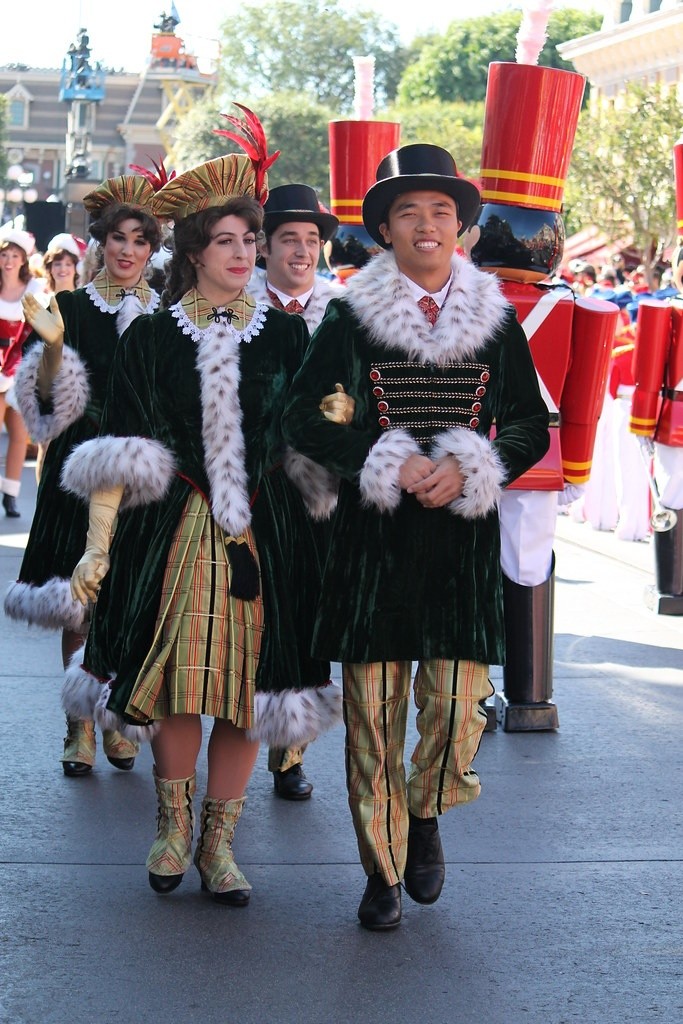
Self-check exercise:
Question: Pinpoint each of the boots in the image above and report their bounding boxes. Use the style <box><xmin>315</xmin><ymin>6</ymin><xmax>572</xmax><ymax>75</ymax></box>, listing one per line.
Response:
<box><xmin>0</xmin><ymin>478</ymin><xmax>20</xmax><ymax>517</ymax></box>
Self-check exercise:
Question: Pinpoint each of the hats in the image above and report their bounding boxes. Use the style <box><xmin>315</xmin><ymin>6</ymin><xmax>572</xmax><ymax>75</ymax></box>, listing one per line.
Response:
<box><xmin>47</xmin><ymin>233</ymin><xmax>83</xmax><ymax>276</ymax></box>
<box><xmin>83</xmin><ymin>153</ymin><xmax>172</xmax><ymax>228</ymax></box>
<box><xmin>256</xmin><ymin>184</ymin><xmax>339</xmax><ymax>271</ymax></box>
<box><xmin>151</xmin><ymin>103</ymin><xmax>280</xmax><ymax>222</ymax></box>
<box><xmin>2</xmin><ymin>229</ymin><xmax>36</xmax><ymax>254</ymax></box>
<box><xmin>362</xmin><ymin>144</ymin><xmax>480</xmax><ymax>251</ymax></box>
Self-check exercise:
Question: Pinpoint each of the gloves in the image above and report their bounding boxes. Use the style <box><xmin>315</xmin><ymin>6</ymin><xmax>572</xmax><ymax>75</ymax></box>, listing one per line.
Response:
<box><xmin>70</xmin><ymin>484</ymin><xmax>124</xmax><ymax>608</ymax></box>
<box><xmin>319</xmin><ymin>382</ymin><xmax>355</xmax><ymax>424</ymax></box>
<box><xmin>21</xmin><ymin>293</ymin><xmax>64</xmax><ymax>402</ymax></box>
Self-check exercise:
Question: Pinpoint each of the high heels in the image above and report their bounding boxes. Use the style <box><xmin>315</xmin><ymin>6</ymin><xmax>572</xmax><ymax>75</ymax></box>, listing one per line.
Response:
<box><xmin>148</xmin><ymin>872</ymin><xmax>184</xmax><ymax>893</ymax></box>
<box><xmin>60</xmin><ymin>761</ymin><xmax>91</xmax><ymax>776</ymax></box>
<box><xmin>193</xmin><ymin>854</ymin><xmax>250</xmax><ymax>906</ymax></box>
<box><xmin>108</xmin><ymin>757</ymin><xmax>134</xmax><ymax>770</ymax></box>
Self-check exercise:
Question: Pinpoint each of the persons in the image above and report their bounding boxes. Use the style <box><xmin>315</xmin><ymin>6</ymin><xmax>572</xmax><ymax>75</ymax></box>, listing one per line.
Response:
<box><xmin>0</xmin><ymin>228</ymin><xmax>87</xmax><ymax>519</ymax></box>
<box><xmin>278</xmin><ymin>144</ymin><xmax>552</xmax><ymax>929</ymax></box>
<box><xmin>3</xmin><ymin>155</ymin><xmax>176</xmax><ymax>774</ymax></box>
<box><xmin>470</xmin><ymin>0</ymin><xmax>618</xmax><ymax>731</ymax></box>
<box><xmin>569</xmin><ymin>239</ymin><xmax>683</xmax><ymax>302</ymax></box>
<box><xmin>325</xmin><ymin>59</ymin><xmax>399</xmax><ymax>287</ymax></box>
<box><xmin>63</xmin><ymin>103</ymin><xmax>357</xmax><ymax>904</ymax></box>
<box><xmin>628</xmin><ymin>144</ymin><xmax>683</xmax><ymax>616</ymax></box>
<box><xmin>248</xmin><ymin>185</ymin><xmax>345</xmax><ymax>801</ymax></box>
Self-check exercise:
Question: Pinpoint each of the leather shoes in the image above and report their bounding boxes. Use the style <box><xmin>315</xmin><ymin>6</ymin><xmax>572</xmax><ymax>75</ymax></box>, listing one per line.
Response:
<box><xmin>275</xmin><ymin>765</ymin><xmax>313</xmax><ymax>800</ymax></box>
<box><xmin>406</xmin><ymin>814</ymin><xmax>445</xmax><ymax>905</ymax></box>
<box><xmin>358</xmin><ymin>874</ymin><xmax>401</xmax><ymax>929</ymax></box>
